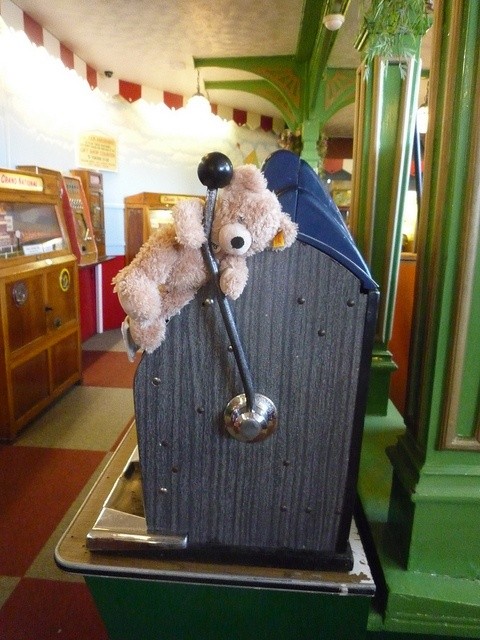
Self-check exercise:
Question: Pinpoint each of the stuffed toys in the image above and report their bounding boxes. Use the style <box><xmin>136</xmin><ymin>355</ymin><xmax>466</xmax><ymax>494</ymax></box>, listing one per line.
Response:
<box><xmin>112</xmin><ymin>163</ymin><xmax>299</xmax><ymax>353</ymax></box>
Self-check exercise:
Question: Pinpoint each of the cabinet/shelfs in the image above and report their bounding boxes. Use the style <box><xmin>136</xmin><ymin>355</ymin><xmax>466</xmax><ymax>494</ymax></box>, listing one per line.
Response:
<box><xmin>0</xmin><ymin>259</ymin><xmax>84</xmax><ymax>441</ymax></box>
